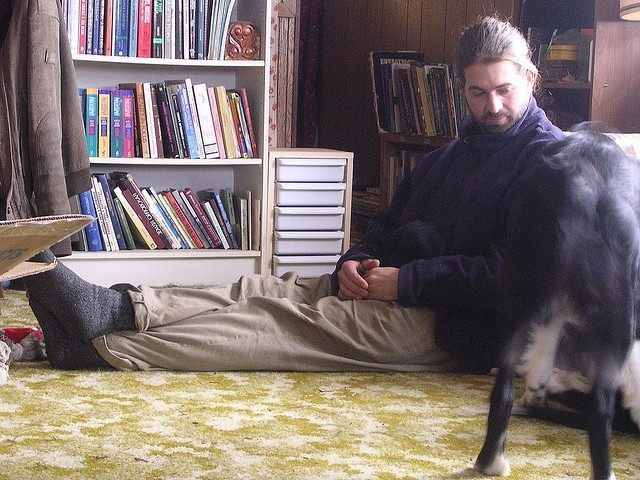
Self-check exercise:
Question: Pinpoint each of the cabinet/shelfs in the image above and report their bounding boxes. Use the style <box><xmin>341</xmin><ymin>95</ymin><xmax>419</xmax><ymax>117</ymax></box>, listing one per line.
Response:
<box><xmin>268</xmin><ymin>147</ymin><xmax>353</xmax><ymax>281</ymax></box>
<box><xmin>523</xmin><ymin>0</ymin><xmax>640</xmax><ymax>132</ymax></box>
<box><xmin>368</xmin><ymin>49</ymin><xmax>458</xmax><ymax>203</ymax></box>
<box><xmin>58</xmin><ymin>0</ymin><xmax>269</xmax><ymax>288</ymax></box>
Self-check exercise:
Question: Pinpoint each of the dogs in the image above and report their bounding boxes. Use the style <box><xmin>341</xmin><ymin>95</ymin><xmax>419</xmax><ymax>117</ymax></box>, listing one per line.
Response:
<box><xmin>472</xmin><ymin>128</ymin><xmax>640</xmax><ymax>480</ymax></box>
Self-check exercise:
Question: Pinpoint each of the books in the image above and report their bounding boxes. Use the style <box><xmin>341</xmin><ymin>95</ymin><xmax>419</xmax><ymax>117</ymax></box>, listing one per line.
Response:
<box><xmin>59</xmin><ymin>0</ymin><xmax>237</xmax><ymax>61</ymax></box>
<box><xmin>352</xmin><ymin>185</ymin><xmax>384</xmax><ymax>225</ymax></box>
<box><xmin>77</xmin><ymin>78</ymin><xmax>259</xmax><ymax>159</ymax></box>
<box><xmin>369</xmin><ymin>48</ymin><xmax>471</xmax><ymax>140</ymax></box>
<box><xmin>68</xmin><ymin>171</ymin><xmax>261</xmax><ymax>250</ymax></box>
<box><xmin>388</xmin><ymin>149</ymin><xmax>423</xmax><ymax>206</ymax></box>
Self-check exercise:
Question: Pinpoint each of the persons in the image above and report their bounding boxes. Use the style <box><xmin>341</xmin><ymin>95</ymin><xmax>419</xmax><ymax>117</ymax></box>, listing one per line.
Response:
<box><xmin>24</xmin><ymin>18</ymin><xmax>568</xmax><ymax>374</ymax></box>
